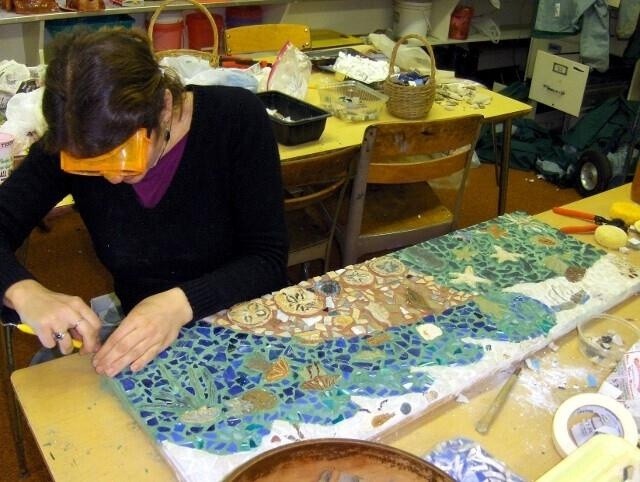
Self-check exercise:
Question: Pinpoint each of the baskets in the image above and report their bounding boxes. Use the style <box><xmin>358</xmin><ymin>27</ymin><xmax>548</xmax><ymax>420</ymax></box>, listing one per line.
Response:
<box><xmin>384</xmin><ymin>33</ymin><xmax>436</xmax><ymax>120</ymax></box>
<box><xmin>146</xmin><ymin>1</ymin><xmax>221</xmax><ymax>69</ymax></box>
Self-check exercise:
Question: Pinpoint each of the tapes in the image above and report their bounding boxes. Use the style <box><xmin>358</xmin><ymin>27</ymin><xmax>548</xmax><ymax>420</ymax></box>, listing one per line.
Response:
<box><xmin>551</xmin><ymin>392</ymin><xmax>638</xmax><ymax>460</ymax></box>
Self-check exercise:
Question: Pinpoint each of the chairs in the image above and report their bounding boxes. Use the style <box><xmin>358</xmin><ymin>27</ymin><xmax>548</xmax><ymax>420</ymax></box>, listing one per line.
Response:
<box><xmin>281</xmin><ymin>113</ymin><xmax>484</xmax><ymax>281</ymax></box>
<box><xmin>224</xmin><ymin>24</ymin><xmax>312</xmax><ymax>57</ymax></box>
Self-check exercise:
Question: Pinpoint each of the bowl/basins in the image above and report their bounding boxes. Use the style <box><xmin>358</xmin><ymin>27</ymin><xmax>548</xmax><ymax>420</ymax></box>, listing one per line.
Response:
<box><xmin>577</xmin><ymin>313</ymin><xmax>640</xmax><ymax>368</ymax></box>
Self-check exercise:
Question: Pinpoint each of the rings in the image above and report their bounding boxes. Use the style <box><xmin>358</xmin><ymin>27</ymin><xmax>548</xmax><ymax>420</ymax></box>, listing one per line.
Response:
<box><xmin>72</xmin><ymin>316</ymin><xmax>84</xmax><ymax>329</ymax></box>
<box><xmin>53</xmin><ymin>332</ymin><xmax>68</xmax><ymax>343</ymax></box>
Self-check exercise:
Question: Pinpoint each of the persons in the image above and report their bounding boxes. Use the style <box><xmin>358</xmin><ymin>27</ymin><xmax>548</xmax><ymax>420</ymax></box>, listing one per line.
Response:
<box><xmin>1</xmin><ymin>29</ymin><xmax>293</xmax><ymax>379</ymax></box>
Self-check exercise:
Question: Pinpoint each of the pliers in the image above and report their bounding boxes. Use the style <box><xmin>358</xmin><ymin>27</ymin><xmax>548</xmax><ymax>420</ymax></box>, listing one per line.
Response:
<box><xmin>553</xmin><ymin>207</ymin><xmax>628</xmax><ymax>234</ymax></box>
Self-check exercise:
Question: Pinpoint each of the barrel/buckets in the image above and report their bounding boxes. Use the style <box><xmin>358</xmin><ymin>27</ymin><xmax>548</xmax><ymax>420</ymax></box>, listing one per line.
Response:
<box><xmin>185</xmin><ymin>11</ymin><xmax>222</xmax><ymax>54</ymax></box>
<box><xmin>225</xmin><ymin>6</ymin><xmax>262</xmax><ymax>28</ymax></box>
<box><xmin>392</xmin><ymin>0</ymin><xmax>433</xmax><ymax>44</ymax></box>
<box><xmin>147</xmin><ymin>10</ymin><xmax>183</xmax><ymax>50</ymax></box>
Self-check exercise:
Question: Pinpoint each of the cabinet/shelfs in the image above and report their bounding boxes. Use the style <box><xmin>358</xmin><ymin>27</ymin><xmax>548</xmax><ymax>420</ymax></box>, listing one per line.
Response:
<box><xmin>0</xmin><ymin>1</ymin><xmax>539</xmax><ymax>48</ymax></box>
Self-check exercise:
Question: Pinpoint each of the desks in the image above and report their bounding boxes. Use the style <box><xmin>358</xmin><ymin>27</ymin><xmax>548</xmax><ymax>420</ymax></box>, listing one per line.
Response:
<box><xmin>0</xmin><ymin>45</ymin><xmax>533</xmax><ymax>217</ymax></box>
<box><xmin>8</xmin><ymin>181</ymin><xmax>639</xmax><ymax>482</ymax></box>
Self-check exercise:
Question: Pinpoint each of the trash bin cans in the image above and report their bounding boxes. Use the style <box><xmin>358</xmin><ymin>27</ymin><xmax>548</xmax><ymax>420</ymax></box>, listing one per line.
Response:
<box><xmin>29</xmin><ymin>292</ymin><xmax>128</xmax><ymax>367</ymax></box>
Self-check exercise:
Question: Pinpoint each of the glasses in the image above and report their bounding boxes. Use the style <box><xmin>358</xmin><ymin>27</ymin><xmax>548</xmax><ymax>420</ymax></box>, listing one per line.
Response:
<box><xmin>59</xmin><ymin>126</ymin><xmax>156</xmax><ymax>180</ymax></box>
